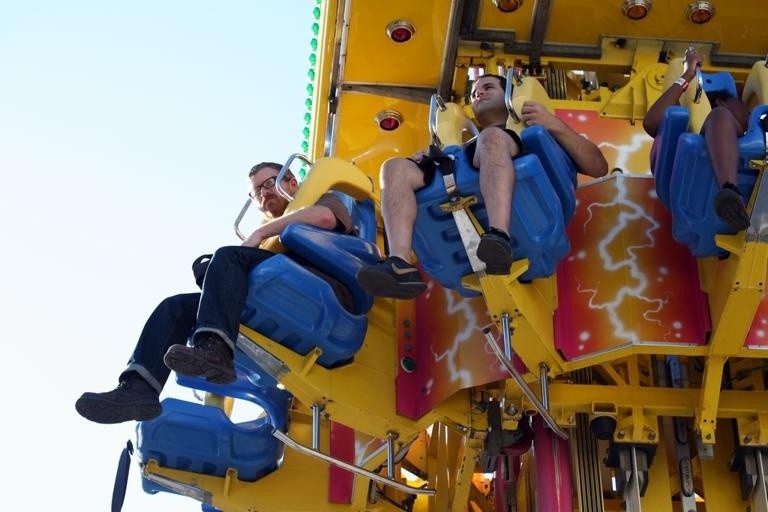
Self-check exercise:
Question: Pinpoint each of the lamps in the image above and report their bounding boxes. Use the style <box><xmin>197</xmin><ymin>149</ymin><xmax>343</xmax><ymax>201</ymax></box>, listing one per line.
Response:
<box><xmin>621</xmin><ymin>0</ymin><xmax>650</xmax><ymax>20</ymax></box>
<box><xmin>489</xmin><ymin>0</ymin><xmax>525</xmax><ymax>12</ymax></box>
<box><xmin>385</xmin><ymin>19</ymin><xmax>416</xmax><ymax>43</ymax></box>
<box><xmin>685</xmin><ymin>1</ymin><xmax>716</xmax><ymax>25</ymax></box>
<box><xmin>372</xmin><ymin>109</ymin><xmax>402</xmax><ymax>131</ymax></box>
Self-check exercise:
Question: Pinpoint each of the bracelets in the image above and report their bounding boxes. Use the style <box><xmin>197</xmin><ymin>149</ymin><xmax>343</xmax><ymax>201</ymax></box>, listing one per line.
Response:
<box><xmin>673</xmin><ymin>78</ymin><xmax>688</xmax><ymax>92</ymax></box>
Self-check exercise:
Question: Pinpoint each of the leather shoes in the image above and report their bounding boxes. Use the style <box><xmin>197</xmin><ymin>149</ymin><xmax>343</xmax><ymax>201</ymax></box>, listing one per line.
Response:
<box><xmin>75</xmin><ymin>375</ymin><xmax>163</xmax><ymax>424</ymax></box>
<box><xmin>164</xmin><ymin>338</ymin><xmax>237</xmax><ymax>385</ymax></box>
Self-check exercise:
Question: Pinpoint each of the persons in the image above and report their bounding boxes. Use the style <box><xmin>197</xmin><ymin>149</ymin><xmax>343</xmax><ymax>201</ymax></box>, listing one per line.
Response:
<box><xmin>357</xmin><ymin>74</ymin><xmax>608</xmax><ymax>301</ymax></box>
<box><xmin>76</xmin><ymin>162</ymin><xmax>358</xmax><ymax>425</ymax></box>
<box><xmin>640</xmin><ymin>48</ymin><xmax>768</xmax><ymax>230</ymax></box>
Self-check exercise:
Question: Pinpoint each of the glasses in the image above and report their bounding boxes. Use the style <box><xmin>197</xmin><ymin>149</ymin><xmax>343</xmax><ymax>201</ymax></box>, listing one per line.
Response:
<box><xmin>248</xmin><ymin>175</ymin><xmax>282</xmax><ymax>199</ymax></box>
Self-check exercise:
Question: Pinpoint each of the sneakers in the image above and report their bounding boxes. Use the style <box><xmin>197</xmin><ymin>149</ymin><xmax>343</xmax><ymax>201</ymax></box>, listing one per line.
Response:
<box><xmin>356</xmin><ymin>257</ymin><xmax>426</xmax><ymax>300</ymax></box>
<box><xmin>476</xmin><ymin>228</ymin><xmax>512</xmax><ymax>276</ymax></box>
<box><xmin>714</xmin><ymin>183</ymin><xmax>751</xmax><ymax>232</ymax></box>
